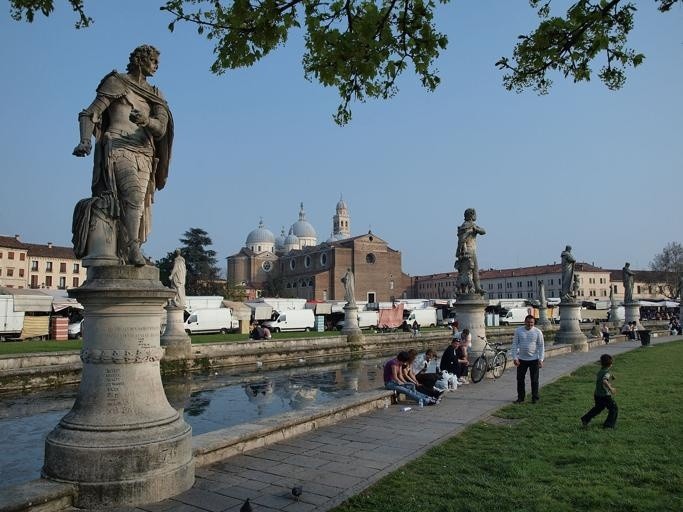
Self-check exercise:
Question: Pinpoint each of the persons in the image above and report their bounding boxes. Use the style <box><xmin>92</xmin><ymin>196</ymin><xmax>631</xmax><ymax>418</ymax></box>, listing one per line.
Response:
<box><xmin>511</xmin><ymin>316</ymin><xmax>545</xmax><ymax>404</ymax></box>
<box><xmin>261</xmin><ymin>325</ymin><xmax>272</xmax><ymax>341</ymax></box>
<box><xmin>561</xmin><ymin>243</ymin><xmax>581</xmax><ymax>300</ymax></box>
<box><xmin>412</xmin><ymin>319</ymin><xmax>422</xmax><ymax>330</ymax></box>
<box><xmin>381</xmin><ymin>330</ymin><xmax>473</xmax><ymax>407</ymax></box>
<box><xmin>537</xmin><ymin>280</ymin><xmax>546</xmax><ymax>302</ymax></box>
<box><xmin>395</xmin><ymin>320</ymin><xmax>412</xmax><ymax>332</ymax></box>
<box><xmin>580</xmin><ymin>354</ymin><xmax>617</xmax><ymax>428</ymax></box>
<box><xmin>167</xmin><ymin>246</ymin><xmax>190</xmax><ymax>309</ymax></box>
<box><xmin>69</xmin><ymin>44</ymin><xmax>176</xmax><ymax>266</ymax></box>
<box><xmin>453</xmin><ymin>208</ymin><xmax>487</xmax><ymax>294</ymax></box>
<box><xmin>247</xmin><ymin>323</ymin><xmax>262</xmax><ymax>339</ymax></box>
<box><xmin>594</xmin><ymin>306</ymin><xmax>683</xmax><ymax>348</ymax></box>
<box><xmin>341</xmin><ymin>265</ymin><xmax>358</xmax><ymax>307</ymax></box>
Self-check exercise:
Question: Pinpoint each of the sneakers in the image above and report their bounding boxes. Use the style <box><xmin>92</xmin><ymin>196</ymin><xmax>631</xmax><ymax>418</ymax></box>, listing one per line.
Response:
<box><xmin>423</xmin><ymin>378</ymin><xmax>469</xmax><ymax>406</ymax></box>
<box><xmin>513</xmin><ymin>400</ymin><xmax>538</xmax><ymax>404</ymax></box>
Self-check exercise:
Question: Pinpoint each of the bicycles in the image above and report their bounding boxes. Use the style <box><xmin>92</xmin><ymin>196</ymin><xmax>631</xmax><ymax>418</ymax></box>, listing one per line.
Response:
<box><xmin>471</xmin><ymin>335</ymin><xmax>508</xmax><ymax>383</ymax></box>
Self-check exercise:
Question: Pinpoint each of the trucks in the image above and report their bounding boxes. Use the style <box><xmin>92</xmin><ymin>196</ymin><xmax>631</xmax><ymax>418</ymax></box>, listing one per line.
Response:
<box><xmin>184</xmin><ymin>308</ymin><xmax>232</xmax><ymax>335</ymax></box>
<box><xmin>261</xmin><ymin>309</ymin><xmax>316</xmax><ymax>333</ymax></box>
<box><xmin>336</xmin><ymin>310</ymin><xmax>379</xmax><ymax>331</ymax></box>
<box><xmin>403</xmin><ymin>308</ymin><xmax>437</xmax><ymax>329</ymax></box>
<box><xmin>498</xmin><ymin>309</ymin><xmax>529</xmax><ymax>325</ymax></box>
